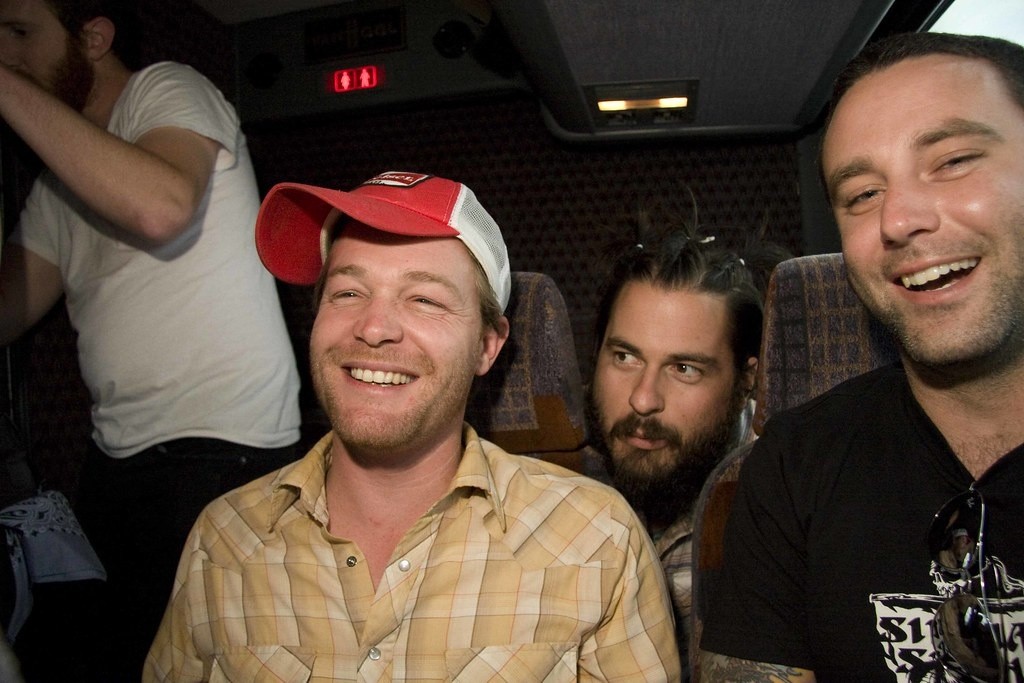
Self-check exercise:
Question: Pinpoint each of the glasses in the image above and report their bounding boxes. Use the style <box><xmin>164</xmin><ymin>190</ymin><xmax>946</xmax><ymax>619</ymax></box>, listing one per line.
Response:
<box><xmin>920</xmin><ymin>481</ymin><xmax>1004</xmax><ymax>683</ymax></box>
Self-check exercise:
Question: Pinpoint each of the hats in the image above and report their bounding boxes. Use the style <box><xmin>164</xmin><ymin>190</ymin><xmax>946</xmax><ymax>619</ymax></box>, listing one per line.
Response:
<box><xmin>254</xmin><ymin>171</ymin><xmax>511</xmax><ymax>320</ymax></box>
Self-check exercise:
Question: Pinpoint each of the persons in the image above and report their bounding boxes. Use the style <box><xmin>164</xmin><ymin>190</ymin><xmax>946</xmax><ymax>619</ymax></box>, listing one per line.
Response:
<box><xmin>0</xmin><ymin>0</ymin><xmax>301</xmax><ymax>683</ymax></box>
<box><xmin>586</xmin><ymin>237</ymin><xmax>764</xmax><ymax>683</ymax></box>
<box><xmin>140</xmin><ymin>170</ymin><xmax>682</xmax><ymax>683</ymax></box>
<box><xmin>698</xmin><ymin>31</ymin><xmax>1024</xmax><ymax>683</ymax></box>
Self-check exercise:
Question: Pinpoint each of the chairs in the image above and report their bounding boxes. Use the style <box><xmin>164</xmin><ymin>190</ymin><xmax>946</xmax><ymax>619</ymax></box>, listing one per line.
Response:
<box><xmin>690</xmin><ymin>252</ymin><xmax>901</xmax><ymax>683</ymax></box>
<box><xmin>461</xmin><ymin>271</ymin><xmax>647</xmax><ymax>529</ymax></box>
